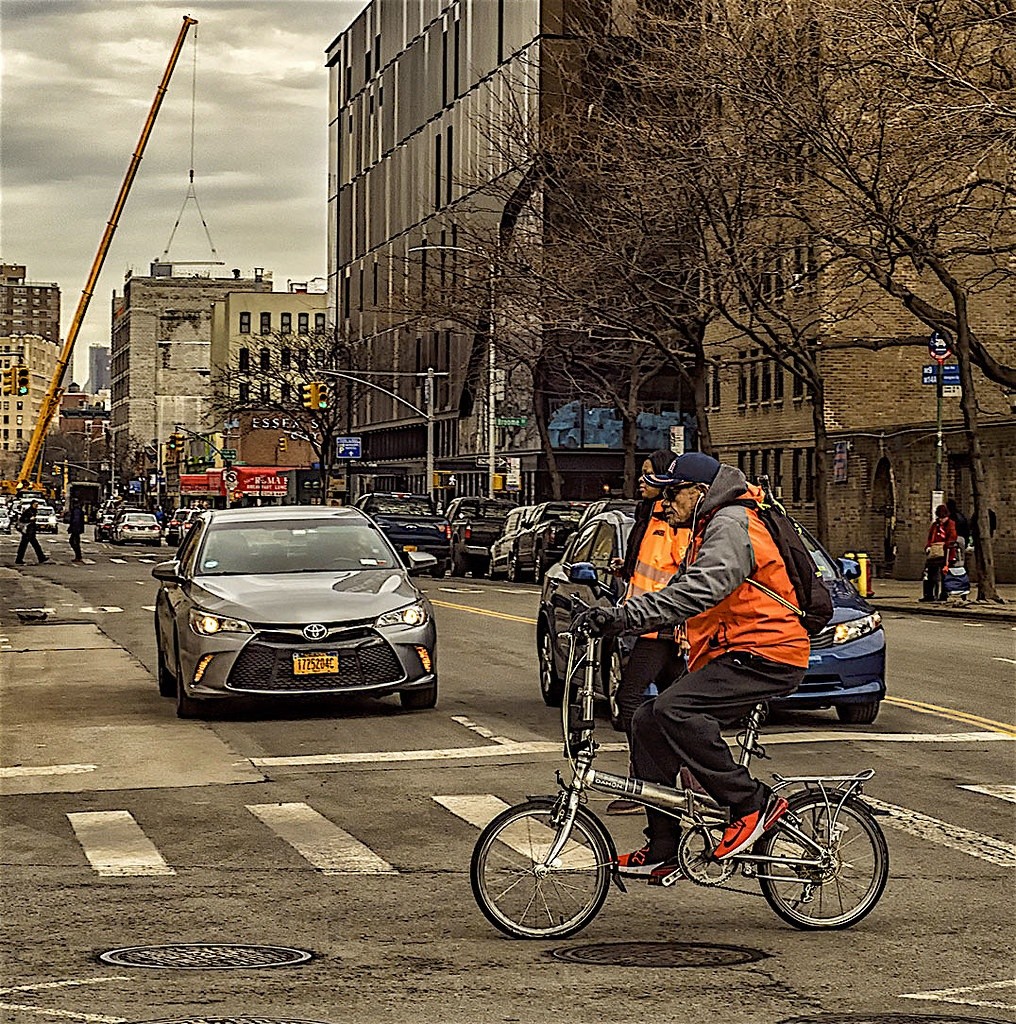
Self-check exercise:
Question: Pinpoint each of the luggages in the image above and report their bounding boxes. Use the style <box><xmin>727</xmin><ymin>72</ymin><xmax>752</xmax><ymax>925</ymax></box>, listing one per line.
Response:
<box><xmin>942</xmin><ymin>544</ymin><xmax>971</xmax><ymax>602</ymax></box>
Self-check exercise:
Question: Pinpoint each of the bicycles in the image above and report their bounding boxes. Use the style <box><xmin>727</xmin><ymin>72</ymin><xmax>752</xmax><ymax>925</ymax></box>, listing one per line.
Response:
<box><xmin>469</xmin><ymin>592</ymin><xmax>893</xmax><ymax>941</ymax></box>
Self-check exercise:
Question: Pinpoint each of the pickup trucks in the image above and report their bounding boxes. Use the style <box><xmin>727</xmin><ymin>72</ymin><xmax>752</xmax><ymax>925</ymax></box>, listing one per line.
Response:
<box><xmin>445</xmin><ymin>494</ymin><xmax>521</xmax><ymax>579</ymax></box>
<box><xmin>352</xmin><ymin>491</ymin><xmax>454</xmax><ymax>579</ymax></box>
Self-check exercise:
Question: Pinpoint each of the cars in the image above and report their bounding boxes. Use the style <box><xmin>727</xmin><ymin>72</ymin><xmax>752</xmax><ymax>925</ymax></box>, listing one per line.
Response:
<box><xmin>93</xmin><ymin>500</ymin><xmax>162</xmax><ymax>546</ymax></box>
<box><xmin>0</xmin><ymin>494</ymin><xmax>59</xmax><ymax>534</ymax></box>
<box><xmin>151</xmin><ymin>504</ymin><xmax>438</xmax><ymax>712</ymax></box>
<box><xmin>535</xmin><ymin>499</ymin><xmax>888</xmax><ymax>732</ymax></box>
<box><xmin>489</xmin><ymin>501</ymin><xmax>594</xmax><ymax>586</ymax></box>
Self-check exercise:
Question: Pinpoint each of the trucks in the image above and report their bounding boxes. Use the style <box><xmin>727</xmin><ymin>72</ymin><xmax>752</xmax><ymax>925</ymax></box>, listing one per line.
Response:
<box><xmin>61</xmin><ymin>480</ymin><xmax>101</xmax><ymax>523</ymax></box>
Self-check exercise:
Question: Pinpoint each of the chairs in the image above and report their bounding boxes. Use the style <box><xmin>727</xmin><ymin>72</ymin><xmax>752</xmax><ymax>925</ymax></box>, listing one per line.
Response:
<box><xmin>305</xmin><ymin>528</ymin><xmax>367</xmax><ymax>570</ymax></box>
<box><xmin>203</xmin><ymin>533</ymin><xmax>255</xmax><ymax>572</ymax></box>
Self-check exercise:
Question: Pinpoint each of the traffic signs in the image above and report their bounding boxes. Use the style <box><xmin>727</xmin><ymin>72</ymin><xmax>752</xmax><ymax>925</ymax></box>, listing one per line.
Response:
<box><xmin>336</xmin><ymin>437</ymin><xmax>362</xmax><ymax>458</ymax></box>
<box><xmin>220</xmin><ymin>449</ymin><xmax>237</xmax><ymax>460</ymax></box>
<box><xmin>476</xmin><ymin>455</ymin><xmax>509</xmax><ymax>468</ymax></box>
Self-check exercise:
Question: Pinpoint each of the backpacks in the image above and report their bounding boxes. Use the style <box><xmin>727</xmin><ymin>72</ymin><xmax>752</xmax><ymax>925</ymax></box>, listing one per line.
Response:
<box><xmin>705</xmin><ymin>497</ymin><xmax>834</xmax><ymax>635</ymax></box>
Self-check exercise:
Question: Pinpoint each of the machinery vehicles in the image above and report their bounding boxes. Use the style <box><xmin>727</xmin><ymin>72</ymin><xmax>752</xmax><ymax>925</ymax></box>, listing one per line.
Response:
<box><xmin>1</xmin><ymin>15</ymin><xmax>198</xmax><ymax>494</ymax></box>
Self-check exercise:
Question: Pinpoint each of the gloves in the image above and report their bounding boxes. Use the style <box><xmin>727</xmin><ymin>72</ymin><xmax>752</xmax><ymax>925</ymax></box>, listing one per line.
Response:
<box><xmin>567</xmin><ymin>609</ymin><xmax>612</xmax><ymax>645</ymax></box>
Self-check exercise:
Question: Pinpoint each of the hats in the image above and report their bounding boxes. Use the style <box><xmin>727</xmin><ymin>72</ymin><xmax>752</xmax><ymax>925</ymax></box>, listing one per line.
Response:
<box><xmin>934</xmin><ymin>505</ymin><xmax>950</xmax><ymax>517</ymax></box>
<box><xmin>643</xmin><ymin>452</ymin><xmax>721</xmax><ymax>488</ymax></box>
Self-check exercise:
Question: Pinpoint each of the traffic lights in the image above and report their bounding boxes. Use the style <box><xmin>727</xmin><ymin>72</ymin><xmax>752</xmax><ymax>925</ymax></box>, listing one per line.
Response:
<box><xmin>197</xmin><ymin>456</ymin><xmax>205</xmax><ymax>466</ymax></box>
<box><xmin>55</xmin><ymin>466</ymin><xmax>61</xmax><ymax>475</ymax></box>
<box><xmin>303</xmin><ymin>381</ymin><xmax>317</xmax><ymax>410</ymax></box>
<box><xmin>16</xmin><ymin>366</ymin><xmax>31</xmax><ymax>395</ymax></box>
<box><xmin>442</xmin><ymin>474</ymin><xmax>456</xmax><ymax>488</ymax></box>
<box><xmin>3</xmin><ymin>368</ymin><xmax>16</xmax><ymax>396</ymax></box>
<box><xmin>187</xmin><ymin>457</ymin><xmax>194</xmax><ymax>466</ymax></box>
<box><xmin>280</xmin><ymin>437</ymin><xmax>288</xmax><ymax>452</ymax></box>
<box><xmin>165</xmin><ymin>433</ymin><xmax>184</xmax><ymax>453</ymax></box>
<box><xmin>317</xmin><ymin>383</ymin><xmax>330</xmax><ymax>409</ymax></box>
<box><xmin>231</xmin><ymin>490</ymin><xmax>243</xmax><ymax>501</ymax></box>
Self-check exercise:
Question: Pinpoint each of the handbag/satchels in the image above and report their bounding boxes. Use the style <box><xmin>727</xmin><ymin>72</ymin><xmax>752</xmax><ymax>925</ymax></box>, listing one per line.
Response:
<box><xmin>930</xmin><ymin>543</ymin><xmax>945</xmax><ymax>559</ymax></box>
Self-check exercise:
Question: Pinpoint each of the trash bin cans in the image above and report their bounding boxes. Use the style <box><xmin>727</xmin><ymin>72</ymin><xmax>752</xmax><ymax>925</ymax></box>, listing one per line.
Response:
<box><xmin>168</xmin><ymin>531</ymin><xmax>180</xmax><ymax>545</ymax></box>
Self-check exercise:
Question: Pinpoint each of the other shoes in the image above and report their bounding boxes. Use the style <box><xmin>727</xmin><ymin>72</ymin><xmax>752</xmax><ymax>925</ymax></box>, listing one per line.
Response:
<box><xmin>608</xmin><ymin>796</ymin><xmax>642</xmax><ymax>812</ymax></box>
<box><xmin>919</xmin><ymin>595</ymin><xmax>933</xmax><ymax>602</ymax></box>
<box><xmin>938</xmin><ymin>596</ymin><xmax>947</xmax><ymax>601</ymax></box>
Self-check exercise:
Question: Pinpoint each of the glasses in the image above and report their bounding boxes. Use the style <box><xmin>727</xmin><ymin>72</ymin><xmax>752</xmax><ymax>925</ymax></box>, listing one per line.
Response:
<box><xmin>663</xmin><ymin>483</ymin><xmax>700</xmax><ymax>501</ymax></box>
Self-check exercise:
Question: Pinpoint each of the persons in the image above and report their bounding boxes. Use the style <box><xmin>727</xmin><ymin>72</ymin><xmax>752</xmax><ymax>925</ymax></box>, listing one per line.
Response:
<box><xmin>918</xmin><ymin>505</ymin><xmax>958</xmax><ymax>601</ymax></box>
<box><xmin>15</xmin><ymin>500</ymin><xmax>50</xmax><ymax>564</ymax></box>
<box><xmin>970</xmin><ymin>508</ymin><xmax>997</xmax><ymax>547</ymax></box>
<box><xmin>571</xmin><ymin>453</ymin><xmax>810</xmax><ymax>879</ymax></box>
<box><xmin>67</xmin><ymin>498</ymin><xmax>84</xmax><ymax>562</ymax></box>
<box><xmin>945</xmin><ymin>497</ymin><xmax>970</xmax><ymax>549</ymax></box>
<box><xmin>606</xmin><ymin>449</ymin><xmax>705</xmax><ymax>815</ymax></box>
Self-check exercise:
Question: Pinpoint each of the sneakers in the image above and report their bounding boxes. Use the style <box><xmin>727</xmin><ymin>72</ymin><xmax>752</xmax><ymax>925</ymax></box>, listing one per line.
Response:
<box><xmin>608</xmin><ymin>837</ymin><xmax>697</xmax><ymax>878</ymax></box>
<box><xmin>713</xmin><ymin>781</ymin><xmax>788</xmax><ymax>860</ymax></box>
